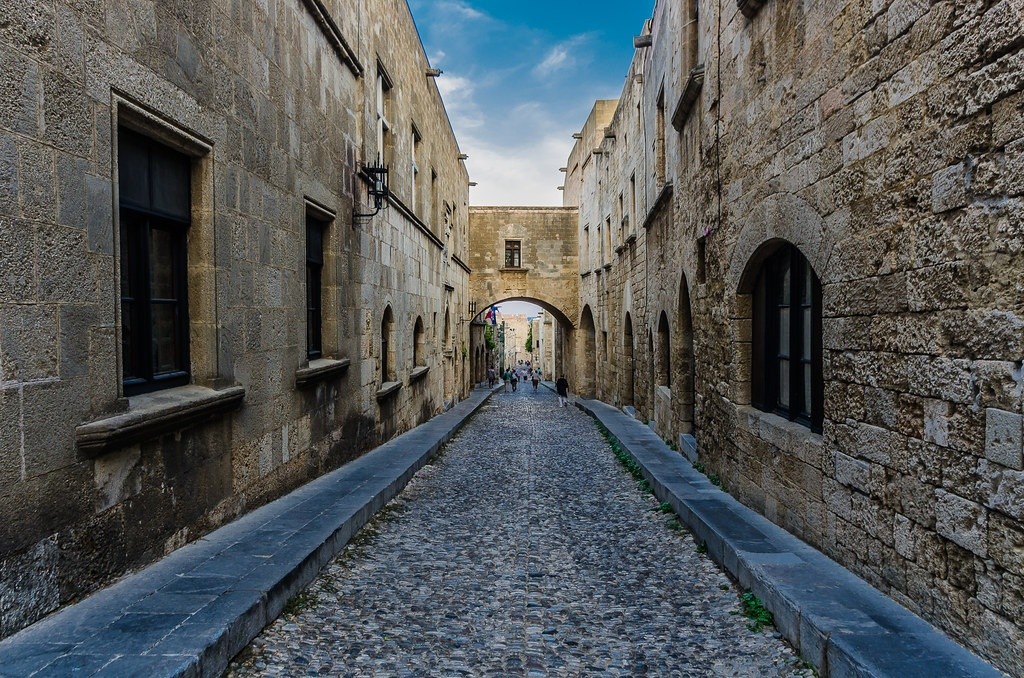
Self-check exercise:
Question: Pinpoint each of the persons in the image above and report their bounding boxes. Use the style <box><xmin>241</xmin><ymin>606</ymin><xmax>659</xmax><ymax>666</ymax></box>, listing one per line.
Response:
<box><xmin>486</xmin><ymin>365</ymin><xmax>496</xmax><ymax>388</ymax></box>
<box><xmin>555</xmin><ymin>374</ymin><xmax>570</xmax><ymax>408</ymax></box>
<box><xmin>503</xmin><ymin>359</ymin><xmax>542</xmax><ymax>393</ymax></box>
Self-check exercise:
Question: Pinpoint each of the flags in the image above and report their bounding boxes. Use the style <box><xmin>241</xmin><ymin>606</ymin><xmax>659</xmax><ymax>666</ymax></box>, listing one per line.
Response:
<box><xmin>485</xmin><ymin>306</ymin><xmax>496</xmax><ymax>325</ymax></box>
